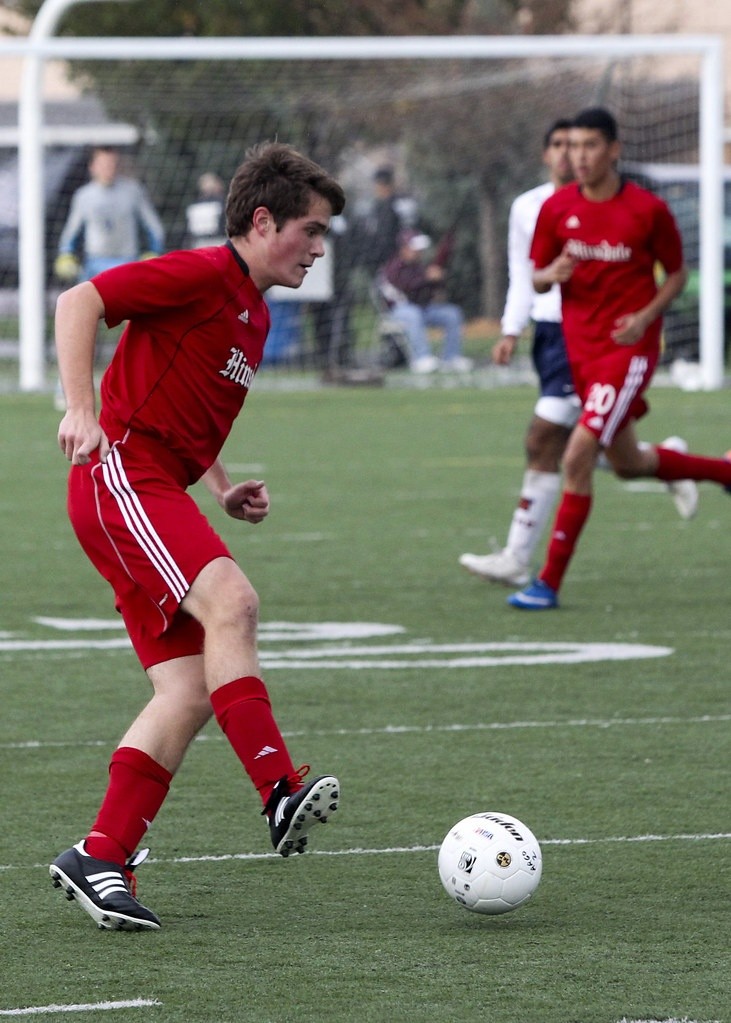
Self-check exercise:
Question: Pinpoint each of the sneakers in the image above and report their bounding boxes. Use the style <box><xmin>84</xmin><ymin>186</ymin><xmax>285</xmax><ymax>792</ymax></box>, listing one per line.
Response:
<box><xmin>459</xmin><ymin>551</ymin><xmax>532</xmax><ymax>586</ymax></box>
<box><xmin>660</xmin><ymin>436</ymin><xmax>699</xmax><ymax>519</ymax></box>
<box><xmin>261</xmin><ymin>774</ymin><xmax>340</xmax><ymax>858</ymax></box>
<box><xmin>506</xmin><ymin>577</ymin><xmax>559</xmax><ymax>610</ymax></box>
<box><xmin>50</xmin><ymin>838</ymin><xmax>161</xmax><ymax>933</ymax></box>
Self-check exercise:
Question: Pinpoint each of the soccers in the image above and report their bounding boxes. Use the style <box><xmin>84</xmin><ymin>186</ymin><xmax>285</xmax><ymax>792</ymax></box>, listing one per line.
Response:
<box><xmin>437</xmin><ymin>809</ymin><xmax>545</xmax><ymax>916</ymax></box>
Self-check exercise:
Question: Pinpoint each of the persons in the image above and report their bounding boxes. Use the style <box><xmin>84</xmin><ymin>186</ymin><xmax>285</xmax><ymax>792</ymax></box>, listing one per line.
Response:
<box><xmin>48</xmin><ymin>105</ymin><xmax>731</xmax><ymax>926</ymax></box>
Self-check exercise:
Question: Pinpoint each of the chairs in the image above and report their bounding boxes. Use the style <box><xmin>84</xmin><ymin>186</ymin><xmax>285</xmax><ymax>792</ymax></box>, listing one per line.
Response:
<box><xmin>369</xmin><ymin>289</ymin><xmax>414</xmax><ymax>375</ymax></box>
